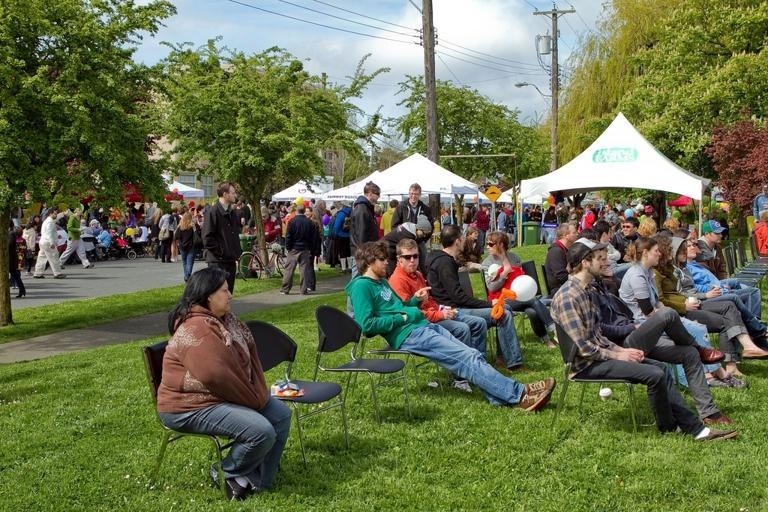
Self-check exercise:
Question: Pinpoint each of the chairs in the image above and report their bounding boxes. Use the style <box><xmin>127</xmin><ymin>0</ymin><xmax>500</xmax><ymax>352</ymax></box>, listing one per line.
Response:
<box><xmin>457</xmin><ymin>258</ymin><xmax>549</xmax><ymax>368</ymax></box>
<box><xmin>353</xmin><ymin>328</ymin><xmax>447</xmax><ymax>408</ymax></box>
<box><xmin>238</xmin><ymin>316</ymin><xmax>351</xmax><ymax>481</ymax></box>
<box><xmin>303</xmin><ymin>305</ymin><xmax>411</xmax><ymax>425</ymax></box>
<box><xmin>544</xmin><ymin>231</ymin><xmax>768</xmax><ymax>443</ymax></box>
<box><xmin>140</xmin><ymin>339</ymin><xmax>240</xmax><ymax>508</ymax></box>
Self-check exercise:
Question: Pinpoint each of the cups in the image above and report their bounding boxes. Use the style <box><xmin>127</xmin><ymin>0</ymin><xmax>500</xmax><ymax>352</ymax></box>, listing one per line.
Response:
<box><xmin>599</xmin><ymin>388</ymin><xmax>613</xmax><ymax>402</ymax></box>
<box><xmin>688</xmin><ymin>296</ymin><xmax>701</xmax><ymax>305</ymax></box>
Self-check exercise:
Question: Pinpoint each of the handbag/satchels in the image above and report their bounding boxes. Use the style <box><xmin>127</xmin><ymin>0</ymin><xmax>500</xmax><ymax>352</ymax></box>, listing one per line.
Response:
<box><xmin>504</xmin><ymin>215</ymin><xmax>510</xmax><ymax>228</ymax></box>
<box><xmin>158</xmin><ymin>228</ymin><xmax>170</xmax><ymax>241</ymax></box>
<box><xmin>342</xmin><ymin>215</ymin><xmax>352</xmax><ymax>232</ymax></box>
<box><xmin>191</xmin><ymin>225</ymin><xmax>206</xmax><ymax>250</ymax></box>
<box><xmin>179</xmin><ymin>240</ymin><xmax>192</xmax><ymax>251</ymax></box>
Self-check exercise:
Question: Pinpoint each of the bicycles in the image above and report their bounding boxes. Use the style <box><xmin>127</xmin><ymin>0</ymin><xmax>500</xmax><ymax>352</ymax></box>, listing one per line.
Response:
<box><xmin>238</xmin><ymin>242</ymin><xmax>287</xmax><ymax>282</ymax></box>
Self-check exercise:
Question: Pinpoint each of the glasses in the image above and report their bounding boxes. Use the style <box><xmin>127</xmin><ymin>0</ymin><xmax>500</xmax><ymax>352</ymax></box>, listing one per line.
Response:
<box><xmin>400</xmin><ymin>254</ymin><xmax>418</xmax><ymax>260</ymax></box>
<box><xmin>486</xmin><ymin>242</ymin><xmax>496</xmax><ymax>247</ymax></box>
<box><xmin>623</xmin><ymin>226</ymin><xmax>633</xmax><ymax>228</ymax></box>
<box><xmin>374</xmin><ymin>193</ymin><xmax>380</xmax><ymax>195</ymax></box>
<box><xmin>376</xmin><ymin>256</ymin><xmax>385</xmax><ymax>261</ymax></box>
<box><xmin>421</xmin><ymin>229</ymin><xmax>428</xmax><ymax>236</ymax></box>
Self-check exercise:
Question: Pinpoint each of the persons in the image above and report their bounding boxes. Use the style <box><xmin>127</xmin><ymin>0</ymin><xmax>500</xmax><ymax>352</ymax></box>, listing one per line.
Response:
<box><xmin>326</xmin><ymin>180</ymin><xmax>768</xmax><ymax>441</ymax></box>
<box><xmin>158</xmin><ymin>267</ymin><xmax>291</xmax><ymax>502</ymax></box>
<box><xmin>7</xmin><ymin>182</ymin><xmax>326</xmax><ymax>297</ymax></box>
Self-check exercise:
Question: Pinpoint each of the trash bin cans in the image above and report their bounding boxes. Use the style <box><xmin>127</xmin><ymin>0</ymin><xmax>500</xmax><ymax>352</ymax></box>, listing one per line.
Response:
<box><xmin>238</xmin><ymin>235</ymin><xmax>258</xmax><ymax>273</ymax></box>
<box><xmin>522</xmin><ymin>221</ymin><xmax>540</xmax><ymax>245</ymax></box>
<box><xmin>542</xmin><ymin>223</ymin><xmax>559</xmax><ymax>244</ymax></box>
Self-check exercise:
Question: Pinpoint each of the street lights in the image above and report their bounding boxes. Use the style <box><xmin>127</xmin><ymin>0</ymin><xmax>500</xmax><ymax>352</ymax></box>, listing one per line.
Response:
<box><xmin>513</xmin><ymin>80</ymin><xmax>560</xmax><ymax>173</ymax></box>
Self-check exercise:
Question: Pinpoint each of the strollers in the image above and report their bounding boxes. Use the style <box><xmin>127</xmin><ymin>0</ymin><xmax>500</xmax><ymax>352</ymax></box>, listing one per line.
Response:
<box><xmin>102</xmin><ymin>230</ymin><xmax>137</xmax><ymax>260</ymax></box>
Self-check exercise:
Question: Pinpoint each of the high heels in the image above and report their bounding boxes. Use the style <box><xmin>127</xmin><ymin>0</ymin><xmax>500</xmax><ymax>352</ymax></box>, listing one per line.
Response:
<box><xmin>16</xmin><ymin>289</ymin><xmax>26</xmax><ymax>299</ymax></box>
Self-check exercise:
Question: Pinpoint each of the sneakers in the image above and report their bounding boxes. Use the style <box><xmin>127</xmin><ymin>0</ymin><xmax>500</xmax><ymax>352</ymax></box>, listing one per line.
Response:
<box><xmin>451</xmin><ymin>380</ymin><xmax>473</xmax><ymax>393</ymax></box>
<box><xmin>741</xmin><ymin>349</ymin><xmax>768</xmax><ymax>359</ymax></box>
<box><xmin>705</xmin><ymin>372</ymin><xmax>746</xmax><ymax>388</ymax></box>
<box><xmin>495</xmin><ymin>356</ymin><xmax>541</xmax><ymax>377</ymax></box>
<box><xmin>512</xmin><ymin>376</ymin><xmax>557</xmax><ymax>412</ymax></box>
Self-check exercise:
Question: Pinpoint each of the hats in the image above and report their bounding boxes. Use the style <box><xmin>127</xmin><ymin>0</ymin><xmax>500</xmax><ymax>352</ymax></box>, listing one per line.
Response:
<box><xmin>624</xmin><ymin>208</ymin><xmax>634</xmax><ymax>219</ymax></box>
<box><xmin>644</xmin><ymin>205</ymin><xmax>653</xmax><ymax>214</ymax></box>
<box><xmin>565</xmin><ymin>236</ymin><xmax>609</xmax><ymax>266</ymax></box>
<box><xmin>702</xmin><ymin>219</ymin><xmax>727</xmax><ymax>235</ymax></box>
<box><xmin>415</xmin><ymin>214</ymin><xmax>432</xmax><ymax>234</ymax></box>
<box><xmin>763</xmin><ymin>184</ymin><xmax>768</xmax><ymax>192</ymax></box>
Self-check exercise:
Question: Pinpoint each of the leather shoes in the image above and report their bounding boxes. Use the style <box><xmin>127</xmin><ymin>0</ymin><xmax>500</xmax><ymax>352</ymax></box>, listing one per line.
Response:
<box><xmin>34</xmin><ymin>275</ymin><xmax>45</xmax><ymax>279</ymax></box>
<box><xmin>703</xmin><ymin>412</ymin><xmax>733</xmax><ymax>426</ymax></box>
<box><xmin>54</xmin><ymin>274</ymin><xmax>66</xmax><ymax>279</ymax></box>
<box><xmin>695</xmin><ymin>428</ymin><xmax>738</xmax><ymax>444</ymax></box>
<box><xmin>216</xmin><ymin>477</ymin><xmax>255</xmax><ymax>502</ymax></box>
<box><xmin>698</xmin><ymin>346</ymin><xmax>726</xmax><ymax>365</ymax></box>
